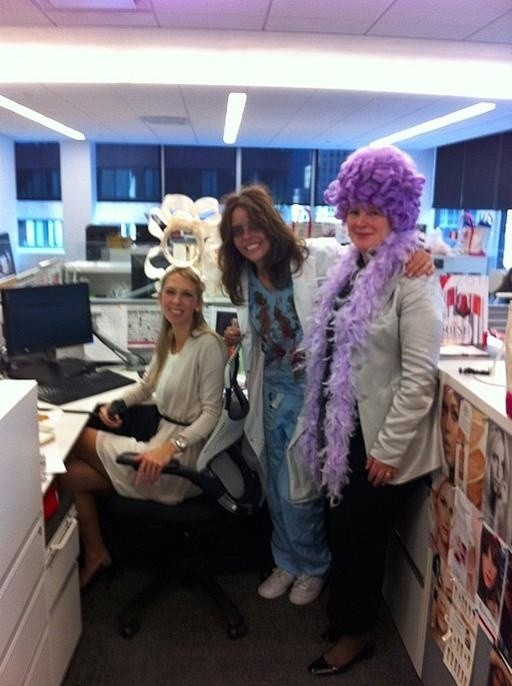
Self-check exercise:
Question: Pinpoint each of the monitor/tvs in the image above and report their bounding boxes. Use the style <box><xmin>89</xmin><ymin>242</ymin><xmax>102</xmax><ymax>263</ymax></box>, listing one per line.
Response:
<box><xmin>1</xmin><ymin>283</ymin><xmax>93</xmax><ymax>383</ymax></box>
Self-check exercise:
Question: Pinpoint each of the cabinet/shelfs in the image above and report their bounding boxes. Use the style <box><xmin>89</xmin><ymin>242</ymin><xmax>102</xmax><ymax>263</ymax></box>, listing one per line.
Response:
<box><xmin>1</xmin><ymin>375</ymin><xmax>85</xmax><ymax>684</ymax></box>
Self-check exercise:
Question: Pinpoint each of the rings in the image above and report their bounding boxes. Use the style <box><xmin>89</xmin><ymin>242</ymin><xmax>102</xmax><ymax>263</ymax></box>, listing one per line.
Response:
<box><xmin>386</xmin><ymin>471</ymin><xmax>391</xmax><ymax>477</ymax></box>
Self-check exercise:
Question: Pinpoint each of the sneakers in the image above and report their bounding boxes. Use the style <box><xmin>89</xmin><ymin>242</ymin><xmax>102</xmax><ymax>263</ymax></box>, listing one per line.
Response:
<box><xmin>257</xmin><ymin>566</ymin><xmax>296</xmax><ymax>600</ymax></box>
<box><xmin>289</xmin><ymin>570</ymin><xmax>327</xmax><ymax>607</ymax></box>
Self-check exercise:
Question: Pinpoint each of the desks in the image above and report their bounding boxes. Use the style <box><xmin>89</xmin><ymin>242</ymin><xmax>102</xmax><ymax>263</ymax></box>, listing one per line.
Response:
<box><xmin>63</xmin><ymin>258</ymin><xmax>132</xmax><ymax>298</ymax></box>
<box><xmin>35</xmin><ymin>363</ymin><xmax>247</xmax><ymax>493</ymax></box>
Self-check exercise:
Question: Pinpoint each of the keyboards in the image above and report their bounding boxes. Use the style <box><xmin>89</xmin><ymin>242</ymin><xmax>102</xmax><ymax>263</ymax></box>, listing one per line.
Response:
<box><xmin>37</xmin><ymin>368</ymin><xmax>136</xmax><ymax>405</ymax></box>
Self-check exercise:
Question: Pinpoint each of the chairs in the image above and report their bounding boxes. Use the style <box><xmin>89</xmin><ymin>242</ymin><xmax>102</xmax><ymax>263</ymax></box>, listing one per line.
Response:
<box><xmin>95</xmin><ymin>340</ymin><xmax>269</xmax><ymax>640</ymax></box>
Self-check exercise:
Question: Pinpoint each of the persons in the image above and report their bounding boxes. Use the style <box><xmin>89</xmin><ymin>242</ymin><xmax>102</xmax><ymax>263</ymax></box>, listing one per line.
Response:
<box><xmin>432</xmin><ymin>382</ymin><xmax>512</xmax><ymax>686</ymax></box>
<box><xmin>306</xmin><ymin>143</ymin><xmax>445</xmax><ymax>678</ymax></box>
<box><xmin>219</xmin><ymin>184</ymin><xmax>436</xmax><ymax>608</ymax></box>
<box><xmin>58</xmin><ymin>264</ymin><xmax>227</xmax><ymax>592</ymax></box>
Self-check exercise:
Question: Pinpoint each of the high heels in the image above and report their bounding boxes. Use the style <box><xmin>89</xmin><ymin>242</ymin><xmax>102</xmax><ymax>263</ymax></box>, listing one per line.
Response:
<box><xmin>80</xmin><ymin>551</ymin><xmax>115</xmax><ymax>608</ymax></box>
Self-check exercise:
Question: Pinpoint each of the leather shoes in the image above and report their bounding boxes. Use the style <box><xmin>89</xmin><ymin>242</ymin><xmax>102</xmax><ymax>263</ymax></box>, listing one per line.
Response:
<box><xmin>306</xmin><ymin>639</ymin><xmax>376</xmax><ymax>678</ymax></box>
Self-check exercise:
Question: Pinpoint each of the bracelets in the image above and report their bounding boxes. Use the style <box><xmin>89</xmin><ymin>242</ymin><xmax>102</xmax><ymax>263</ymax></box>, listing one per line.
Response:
<box><xmin>174</xmin><ymin>435</ymin><xmax>188</xmax><ymax>452</ymax></box>
